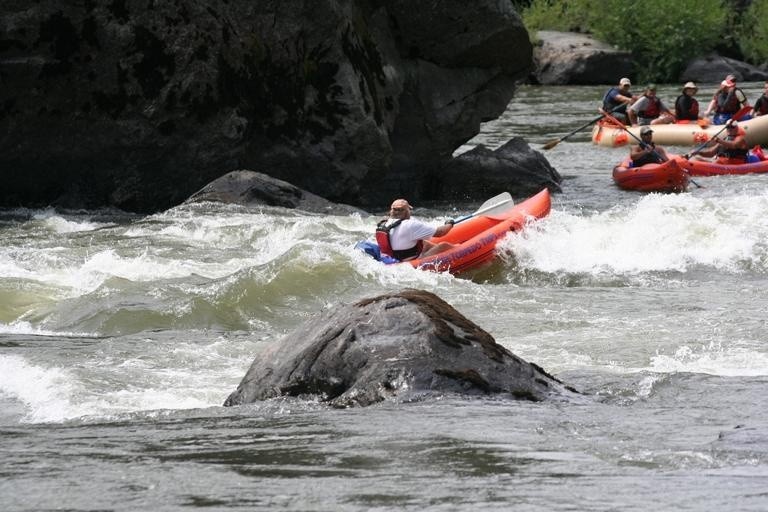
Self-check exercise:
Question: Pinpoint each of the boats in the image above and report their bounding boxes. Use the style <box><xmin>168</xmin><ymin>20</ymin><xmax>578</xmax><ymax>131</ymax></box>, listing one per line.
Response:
<box><xmin>353</xmin><ymin>187</ymin><xmax>551</xmax><ymax>276</ymax></box>
<box><xmin>591</xmin><ymin>110</ymin><xmax>768</xmax><ymax>149</ymax></box>
<box><xmin>677</xmin><ymin>153</ymin><xmax>768</xmax><ymax>174</ymax></box>
<box><xmin>612</xmin><ymin>152</ymin><xmax>690</xmax><ymax>193</ymax></box>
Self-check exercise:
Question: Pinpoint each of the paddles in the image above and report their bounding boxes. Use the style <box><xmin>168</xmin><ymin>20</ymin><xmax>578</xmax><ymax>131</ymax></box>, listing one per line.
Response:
<box><xmin>598</xmin><ymin>107</ymin><xmax>702</xmax><ymax>189</ymax></box>
<box><xmin>689</xmin><ymin>105</ymin><xmax>752</xmax><ymax>157</ymax></box>
<box><xmin>453</xmin><ymin>193</ymin><xmax>514</xmax><ymax>224</ymax></box>
<box><xmin>543</xmin><ymin>101</ymin><xmax>630</xmax><ymax>150</ymax></box>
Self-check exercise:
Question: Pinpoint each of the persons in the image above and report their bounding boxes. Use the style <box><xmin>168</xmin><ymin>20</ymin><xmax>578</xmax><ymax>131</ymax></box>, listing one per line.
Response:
<box><xmin>752</xmin><ymin>81</ymin><xmax>768</xmax><ymax>116</ymax></box>
<box><xmin>628</xmin><ymin>84</ymin><xmax>674</xmax><ymax>125</ymax></box>
<box><xmin>704</xmin><ymin>72</ymin><xmax>754</xmax><ymax>123</ymax></box>
<box><xmin>601</xmin><ymin>77</ymin><xmax>638</xmax><ymax>125</ymax></box>
<box><xmin>673</xmin><ymin>82</ymin><xmax>699</xmax><ymax>121</ymax></box>
<box><xmin>692</xmin><ymin>118</ymin><xmax>749</xmax><ymax>166</ymax></box>
<box><xmin>376</xmin><ymin>200</ymin><xmax>460</xmax><ymax>260</ymax></box>
<box><xmin>629</xmin><ymin>126</ymin><xmax>671</xmax><ymax>166</ymax></box>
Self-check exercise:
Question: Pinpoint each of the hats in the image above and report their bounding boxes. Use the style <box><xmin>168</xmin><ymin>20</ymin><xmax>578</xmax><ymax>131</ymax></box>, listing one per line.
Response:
<box><xmin>647</xmin><ymin>83</ymin><xmax>657</xmax><ymax>90</ymax></box>
<box><xmin>684</xmin><ymin>82</ymin><xmax>697</xmax><ymax>89</ymax></box>
<box><xmin>725</xmin><ymin>75</ymin><xmax>737</xmax><ymax>81</ymax></box>
<box><xmin>620</xmin><ymin>77</ymin><xmax>631</xmax><ymax>87</ymax></box>
<box><xmin>726</xmin><ymin>119</ymin><xmax>738</xmax><ymax>127</ymax></box>
<box><xmin>391</xmin><ymin>199</ymin><xmax>413</xmax><ymax>212</ymax></box>
<box><xmin>640</xmin><ymin>125</ymin><xmax>653</xmax><ymax>138</ymax></box>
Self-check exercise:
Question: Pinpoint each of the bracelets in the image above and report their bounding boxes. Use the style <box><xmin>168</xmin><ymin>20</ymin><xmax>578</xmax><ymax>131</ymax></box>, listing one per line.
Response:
<box><xmin>445</xmin><ymin>219</ymin><xmax>454</xmax><ymax>227</ymax></box>
